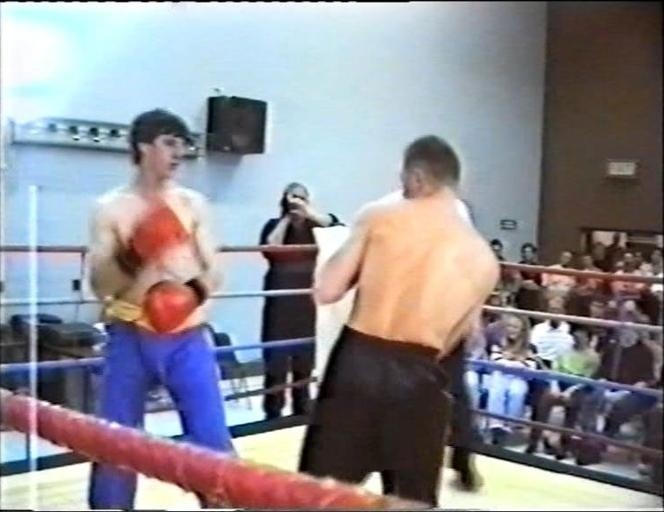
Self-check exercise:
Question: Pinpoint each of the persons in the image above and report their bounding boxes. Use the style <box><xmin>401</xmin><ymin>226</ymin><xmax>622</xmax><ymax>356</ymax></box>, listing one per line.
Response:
<box><xmin>89</xmin><ymin>109</ymin><xmax>238</xmax><ymax>512</ymax></box>
<box><xmin>255</xmin><ymin>182</ymin><xmax>349</xmax><ymax>421</ymax></box>
<box><xmin>467</xmin><ymin>233</ymin><xmax>664</xmax><ymax>480</ymax></box>
<box><xmin>299</xmin><ymin>136</ymin><xmax>499</xmax><ymax>512</ymax></box>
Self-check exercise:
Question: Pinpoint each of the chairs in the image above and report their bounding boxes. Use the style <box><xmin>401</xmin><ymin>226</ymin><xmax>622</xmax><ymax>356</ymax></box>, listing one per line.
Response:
<box><xmin>217</xmin><ymin>329</ymin><xmax>265</xmax><ymax>411</ymax></box>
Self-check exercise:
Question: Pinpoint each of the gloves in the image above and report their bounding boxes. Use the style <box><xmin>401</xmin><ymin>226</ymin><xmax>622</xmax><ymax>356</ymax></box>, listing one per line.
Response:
<box><xmin>116</xmin><ymin>206</ymin><xmax>189</xmax><ymax>277</ymax></box>
<box><xmin>144</xmin><ymin>278</ymin><xmax>208</xmax><ymax>334</ymax></box>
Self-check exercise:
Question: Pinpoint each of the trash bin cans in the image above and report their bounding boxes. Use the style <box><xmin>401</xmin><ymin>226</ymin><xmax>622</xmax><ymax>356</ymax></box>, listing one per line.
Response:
<box><xmin>1</xmin><ymin>314</ymin><xmax>106</xmax><ymax>409</ymax></box>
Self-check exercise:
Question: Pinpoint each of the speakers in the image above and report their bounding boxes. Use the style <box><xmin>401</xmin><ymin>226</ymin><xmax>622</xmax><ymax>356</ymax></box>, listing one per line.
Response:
<box><xmin>206</xmin><ymin>96</ymin><xmax>266</xmax><ymax>153</ymax></box>
